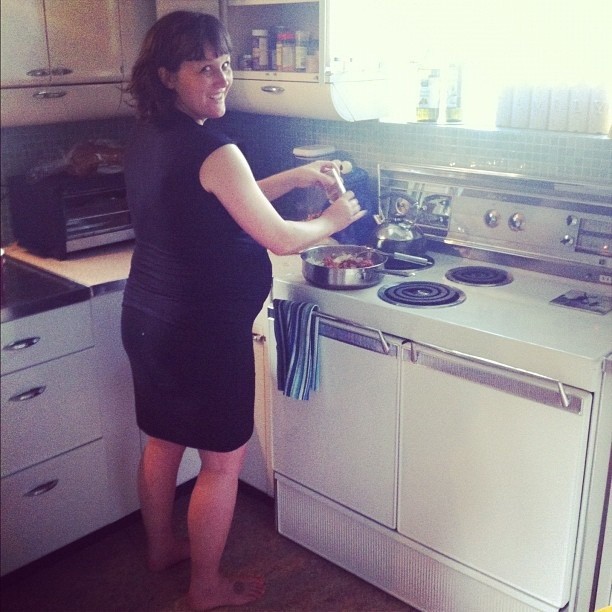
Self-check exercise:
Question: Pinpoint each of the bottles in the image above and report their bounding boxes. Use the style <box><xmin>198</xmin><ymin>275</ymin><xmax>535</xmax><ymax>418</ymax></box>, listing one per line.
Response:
<box><xmin>250</xmin><ymin>29</ymin><xmax>270</xmax><ymax>70</ymax></box>
<box><xmin>295</xmin><ymin>45</ymin><xmax>309</xmax><ymax>68</ymax></box>
<box><xmin>276</xmin><ymin>32</ymin><xmax>296</xmax><ymax>70</ymax></box>
<box><xmin>241</xmin><ymin>55</ymin><xmax>250</xmax><ymax>69</ymax></box>
<box><xmin>295</xmin><ymin>31</ymin><xmax>310</xmax><ymax>49</ymax></box>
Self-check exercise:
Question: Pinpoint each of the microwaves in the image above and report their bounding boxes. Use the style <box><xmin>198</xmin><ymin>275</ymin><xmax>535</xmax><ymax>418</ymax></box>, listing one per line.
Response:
<box><xmin>9</xmin><ymin>178</ymin><xmax>147</xmax><ymax>262</ymax></box>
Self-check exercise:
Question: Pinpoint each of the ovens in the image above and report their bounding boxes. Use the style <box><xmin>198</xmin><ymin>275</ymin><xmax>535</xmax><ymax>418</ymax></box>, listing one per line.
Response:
<box><xmin>264</xmin><ymin>296</ymin><xmax>611</xmax><ymax>612</ymax></box>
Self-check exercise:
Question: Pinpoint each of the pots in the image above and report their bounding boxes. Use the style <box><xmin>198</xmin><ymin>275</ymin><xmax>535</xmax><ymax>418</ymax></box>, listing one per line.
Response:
<box><xmin>301</xmin><ymin>246</ymin><xmax>414</xmax><ymax>289</ymax></box>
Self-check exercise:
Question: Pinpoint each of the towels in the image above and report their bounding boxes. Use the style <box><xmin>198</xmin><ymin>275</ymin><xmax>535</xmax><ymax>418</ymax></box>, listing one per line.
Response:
<box><xmin>273</xmin><ymin>299</ymin><xmax>320</xmax><ymax>400</ymax></box>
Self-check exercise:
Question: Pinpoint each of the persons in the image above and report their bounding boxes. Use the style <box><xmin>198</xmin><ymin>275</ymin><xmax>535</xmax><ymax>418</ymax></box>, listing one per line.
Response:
<box><xmin>120</xmin><ymin>10</ymin><xmax>368</xmax><ymax>611</ymax></box>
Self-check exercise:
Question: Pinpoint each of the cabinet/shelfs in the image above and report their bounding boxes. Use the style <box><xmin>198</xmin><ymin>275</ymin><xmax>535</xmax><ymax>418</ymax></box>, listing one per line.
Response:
<box><xmin>221</xmin><ymin>0</ymin><xmax>379</xmax><ymax>124</ymax></box>
<box><xmin>238</xmin><ymin>303</ymin><xmax>275</xmax><ymax>500</ymax></box>
<box><xmin>0</xmin><ymin>0</ymin><xmax>158</xmax><ymax>130</ymax></box>
<box><xmin>267</xmin><ymin>278</ymin><xmax>402</xmax><ymax>599</ymax></box>
<box><xmin>395</xmin><ymin>310</ymin><xmax>602</xmax><ymax>612</ymax></box>
<box><xmin>0</xmin><ymin>289</ymin><xmax>112</xmax><ymax>578</ymax></box>
<box><xmin>93</xmin><ymin>278</ymin><xmax>202</xmax><ymax>526</ymax></box>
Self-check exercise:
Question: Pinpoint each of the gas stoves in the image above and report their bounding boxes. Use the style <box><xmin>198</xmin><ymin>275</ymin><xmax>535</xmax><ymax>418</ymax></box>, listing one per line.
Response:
<box><xmin>255</xmin><ymin>245</ymin><xmax>611</xmax><ymax>363</ymax></box>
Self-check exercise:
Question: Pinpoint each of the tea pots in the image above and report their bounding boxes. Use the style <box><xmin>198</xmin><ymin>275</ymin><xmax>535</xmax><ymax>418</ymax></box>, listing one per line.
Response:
<box><xmin>369</xmin><ymin>192</ymin><xmax>426</xmax><ymax>267</ymax></box>
